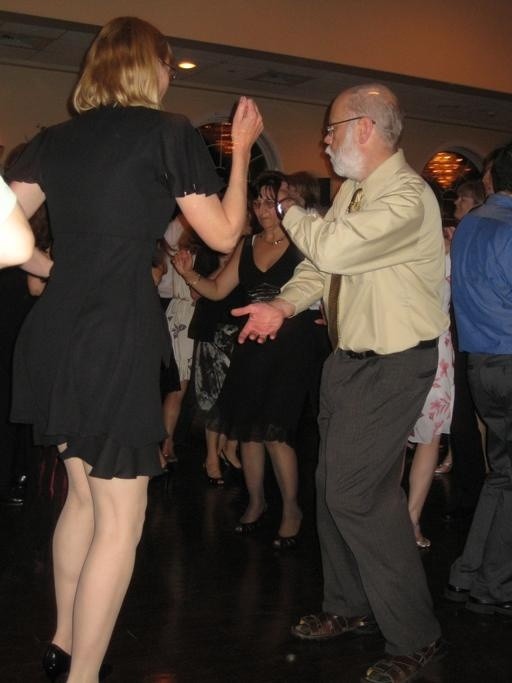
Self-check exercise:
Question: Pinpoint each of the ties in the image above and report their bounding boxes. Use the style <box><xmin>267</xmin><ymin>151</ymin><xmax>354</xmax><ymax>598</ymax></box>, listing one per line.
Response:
<box><xmin>328</xmin><ymin>188</ymin><xmax>363</xmax><ymax>353</ymax></box>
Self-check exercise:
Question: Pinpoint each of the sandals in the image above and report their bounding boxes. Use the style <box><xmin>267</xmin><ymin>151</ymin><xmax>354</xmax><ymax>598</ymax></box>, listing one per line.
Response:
<box><xmin>272</xmin><ymin>511</ymin><xmax>305</xmax><ymax>550</ymax></box>
<box><xmin>202</xmin><ymin>448</ymin><xmax>244</xmax><ymax>488</ymax></box>
<box><xmin>234</xmin><ymin>500</ymin><xmax>269</xmax><ymax>534</ymax></box>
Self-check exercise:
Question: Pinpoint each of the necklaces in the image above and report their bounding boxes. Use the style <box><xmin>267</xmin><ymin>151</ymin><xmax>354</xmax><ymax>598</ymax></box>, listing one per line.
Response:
<box><xmin>262</xmin><ymin>235</ymin><xmax>288</xmax><ymax>246</ymax></box>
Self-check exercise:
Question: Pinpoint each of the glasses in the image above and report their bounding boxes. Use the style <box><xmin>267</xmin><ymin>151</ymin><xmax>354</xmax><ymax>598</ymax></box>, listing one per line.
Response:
<box><xmin>159</xmin><ymin>58</ymin><xmax>177</xmax><ymax>79</ymax></box>
<box><xmin>248</xmin><ymin>198</ymin><xmax>275</xmax><ymax>210</ymax></box>
<box><xmin>325</xmin><ymin>115</ymin><xmax>376</xmax><ymax>136</ymax></box>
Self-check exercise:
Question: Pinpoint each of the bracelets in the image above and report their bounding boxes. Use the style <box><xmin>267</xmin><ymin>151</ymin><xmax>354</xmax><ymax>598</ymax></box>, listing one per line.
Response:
<box><xmin>186</xmin><ymin>273</ymin><xmax>200</xmax><ymax>285</ymax></box>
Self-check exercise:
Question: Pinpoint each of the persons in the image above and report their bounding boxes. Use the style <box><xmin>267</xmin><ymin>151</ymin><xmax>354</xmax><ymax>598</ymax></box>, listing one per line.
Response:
<box><xmin>0</xmin><ymin>16</ymin><xmax>512</xmax><ymax>683</ymax></box>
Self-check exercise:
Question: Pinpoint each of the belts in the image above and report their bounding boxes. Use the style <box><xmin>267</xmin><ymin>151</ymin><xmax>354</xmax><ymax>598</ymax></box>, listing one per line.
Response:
<box><xmin>347</xmin><ymin>338</ymin><xmax>436</xmax><ymax>360</ymax></box>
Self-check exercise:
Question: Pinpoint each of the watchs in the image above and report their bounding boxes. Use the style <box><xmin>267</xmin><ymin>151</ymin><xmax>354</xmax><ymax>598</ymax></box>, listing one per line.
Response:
<box><xmin>277</xmin><ymin>197</ymin><xmax>292</xmax><ymax>219</ymax></box>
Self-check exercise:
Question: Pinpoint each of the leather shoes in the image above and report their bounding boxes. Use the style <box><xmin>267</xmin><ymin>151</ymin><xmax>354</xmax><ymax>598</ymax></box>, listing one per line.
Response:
<box><xmin>44</xmin><ymin>643</ymin><xmax>111</xmax><ymax>679</ymax></box>
<box><xmin>434</xmin><ymin>461</ymin><xmax>452</xmax><ymax>475</ymax></box>
<box><xmin>416</xmin><ymin>536</ymin><xmax>432</xmax><ymax>549</ymax></box>
<box><xmin>447</xmin><ymin>584</ymin><xmax>512</xmax><ymax>618</ymax></box>
<box><xmin>362</xmin><ymin>635</ymin><xmax>448</xmax><ymax>683</ymax></box>
<box><xmin>1</xmin><ymin>489</ymin><xmax>24</xmax><ymax>507</ymax></box>
<box><xmin>159</xmin><ymin>438</ymin><xmax>178</xmax><ymax>469</ymax></box>
<box><xmin>291</xmin><ymin>610</ymin><xmax>379</xmax><ymax>641</ymax></box>
